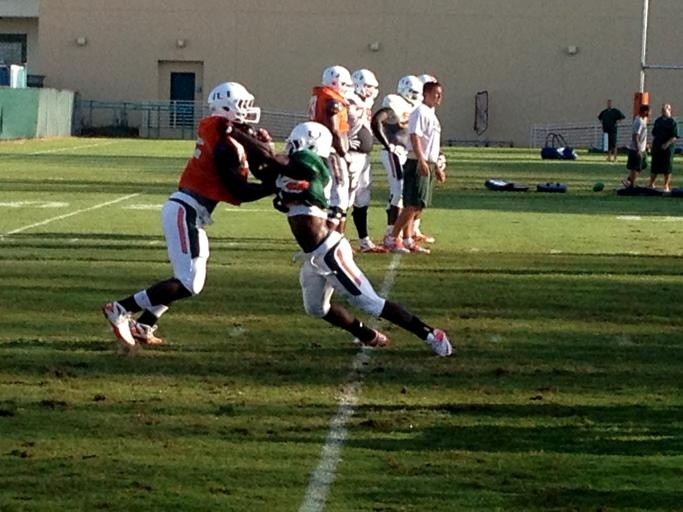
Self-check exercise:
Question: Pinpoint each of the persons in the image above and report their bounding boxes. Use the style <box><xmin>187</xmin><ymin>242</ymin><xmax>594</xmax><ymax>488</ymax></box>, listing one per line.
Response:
<box><xmin>621</xmin><ymin>104</ymin><xmax>649</xmax><ymax>191</ymax></box>
<box><xmin>597</xmin><ymin>99</ymin><xmax>626</xmax><ymax>163</ymax></box>
<box><xmin>214</xmin><ymin>119</ymin><xmax>454</xmax><ymax>359</ymax></box>
<box><xmin>305</xmin><ymin>64</ymin><xmax>446</xmax><ymax>256</ymax></box>
<box><xmin>101</xmin><ymin>83</ymin><xmax>292</xmax><ymax>348</ymax></box>
<box><xmin>646</xmin><ymin>103</ymin><xmax>680</xmax><ymax>193</ymax></box>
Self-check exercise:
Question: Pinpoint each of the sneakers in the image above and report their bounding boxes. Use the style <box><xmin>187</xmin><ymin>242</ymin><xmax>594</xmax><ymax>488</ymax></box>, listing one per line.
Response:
<box><xmin>663</xmin><ymin>188</ymin><xmax>671</xmax><ymax>193</ymax></box>
<box><xmin>648</xmin><ymin>186</ymin><xmax>656</xmax><ymax>190</ymax></box>
<box><xmin>383</xmin><ymin>235</ymin><xmax>410</xmax><ymax>254</ymax></box>
<box><xmin>358</xmin><ymin>243</ymin><xmax>389</xmax><ymax>253</ymax></box>
<box><xmin>411</xmin><ymin>231</ymin><xmax>435</xmax><ymax>244</ymax></box>
<box><xmin>424</xmin><ymin>328</ymin><xmax>452</xmax><ymax>357</ymax></box>
<box><xmin>402</xmin><ymin>238</ymin><xmax>430</xmax><ymax>254</ymax></box>
<box><xmin>352</xmin><ymin>329</ymin><xmax>389</xmax><ymax>347</ymax></box>
<box><xmin>100</xmin><ymin>301</ymin><xmax>135</xmax><ymax>348</ymax></box>
<box><xmin>128</xmin><ymin>320</ymin><xmax>162</xmax><ymax>345</ymax></box>
<box><xmin>621</xmin><ymin>179</ymin><xmax>631</xmax><ymax>188</ymax></box>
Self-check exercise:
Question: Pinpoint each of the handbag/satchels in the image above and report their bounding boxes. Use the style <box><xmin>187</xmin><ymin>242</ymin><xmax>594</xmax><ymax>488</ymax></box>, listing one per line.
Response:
<box><xmin>540</xmin><ymin>133</ymin><xmax>576</xmax><ymax>160</ymax></box>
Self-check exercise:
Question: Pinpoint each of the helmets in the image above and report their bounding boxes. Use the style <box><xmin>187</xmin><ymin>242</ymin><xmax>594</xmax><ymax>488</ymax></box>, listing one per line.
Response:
<box><xmin>207</xmin><ymin>82</ymin><xmax>260</xmax><ymax>124</ymax></box>
<box><xmin>282</xmin><ymin>122</ymin><xmax>332</xmax><ymax>159</ymax></box>
<box><xmin>350</xmin><ymin>69</ymin><xmax>379</xmax><ymax>99</ymax></box>
<box><xmin>321</xmin><ymin>66</ymin><xmax>353</xmax><ymax>99</ymax></box>
<box><xmin>416</xmin><ymin>75</ymin><xmax>437</xmax><ymax>87</ymax></box>
<box><xmin>396</xmin><ymin>76</ymin><xmax>423</xmax><ymax>104</ymax></box>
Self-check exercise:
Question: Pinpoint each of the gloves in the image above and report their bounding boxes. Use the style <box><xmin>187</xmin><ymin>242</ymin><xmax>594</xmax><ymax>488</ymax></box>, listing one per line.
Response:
<box><xmin>275</xmin><ymin>173</ymin><xmax>309</xmax><ymax>193</ymax></box>
<box><xmin>436</xmin><ymin>153</ymin><xmax>447</xmax><ymax>171</ymax></box>
<box><xmin>387</xmin><ymin>143</ymin><xmax>408</xmax><ymax>165</ymax></box>
<box><xmin>348</xmin><ymin>135</ymin><xmax>361</xmax><ymax>150</ymax></box>
<box><xmin>255</xmin><ymin>127</ymin><xmax>272</xmax><ymax>143</ymax></box>
<box><xmin>343</xmin><ymin>153</ymin><xmax>360</xmax><ymax>193</ymax></box>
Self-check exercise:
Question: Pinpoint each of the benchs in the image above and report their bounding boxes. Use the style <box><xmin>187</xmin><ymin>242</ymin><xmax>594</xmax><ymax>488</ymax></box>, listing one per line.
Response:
<box><xmin>446</xmin><ymin>137</ymin><xmax>514</xmax><ymax>148</ymax></box>
